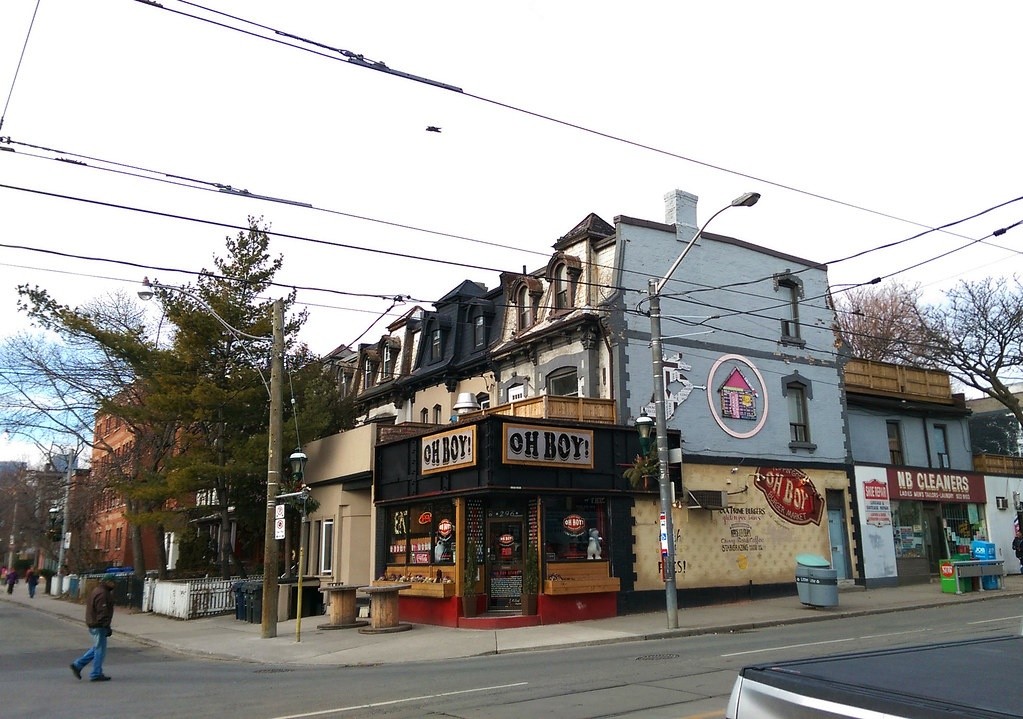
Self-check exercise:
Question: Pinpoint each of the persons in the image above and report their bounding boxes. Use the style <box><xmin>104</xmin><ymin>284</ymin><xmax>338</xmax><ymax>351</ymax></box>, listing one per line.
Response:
<box><xmin>25</xmin><ymin>565</ymin><xmax>40</xmax><ymax>598</ymax></box>
<box><xmin>4</xmin><ymin>567</ymin><xmax>19</xmax><ymax>595</ymax></box>
<box><xmin>69</xmin><ymin>573</ymin><xmax>115</xmax><ymax>681</ymax></box>
<box><xmin>1011</xmin><ymin>530</ymin><xmax>1023</xmax><ymax>573</ymax></box>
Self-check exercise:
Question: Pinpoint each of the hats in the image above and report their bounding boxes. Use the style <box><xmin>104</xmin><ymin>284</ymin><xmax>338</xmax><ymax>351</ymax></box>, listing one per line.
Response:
<box><xmin>102</xmin><ymin>573</ymin><xmax>114</xmax><ymax>582</ymax></box>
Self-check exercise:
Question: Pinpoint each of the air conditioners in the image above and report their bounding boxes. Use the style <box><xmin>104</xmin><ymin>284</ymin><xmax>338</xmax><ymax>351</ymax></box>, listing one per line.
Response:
<box><xmin>686</xmin><ymin>490</ymin><xmax>728</xmax><ymax>510</ymax></box>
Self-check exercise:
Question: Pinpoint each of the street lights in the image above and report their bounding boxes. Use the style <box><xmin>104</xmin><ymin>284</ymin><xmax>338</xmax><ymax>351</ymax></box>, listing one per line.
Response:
<box><xmin>135</xmin><ymin>276</ymin><xmax>285</xmax><ymax>636</ymax></box>
<box><xmin>646</xmin><ymin>187</ymin><xmax>761</xmax><ymax>631</ymax></box>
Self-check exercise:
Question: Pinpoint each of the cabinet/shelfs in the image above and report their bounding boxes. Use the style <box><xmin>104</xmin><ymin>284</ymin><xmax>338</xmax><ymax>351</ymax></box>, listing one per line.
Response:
<box><xmin>392</xmin><ymin>537</ymin><xmax>438</xmax><ymax>563</ymax></box>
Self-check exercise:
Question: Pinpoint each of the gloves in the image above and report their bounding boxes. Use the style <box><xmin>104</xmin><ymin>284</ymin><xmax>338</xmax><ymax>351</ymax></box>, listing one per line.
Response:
<box><xmin>105</xmin><ymin>629</ymin><xmax>112</xmax><ymax>637</ymax></box>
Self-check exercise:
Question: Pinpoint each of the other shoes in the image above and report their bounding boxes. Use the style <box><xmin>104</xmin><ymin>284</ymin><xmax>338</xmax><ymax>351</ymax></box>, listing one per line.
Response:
<box><xmin>69</xmin><ymin>662</ymin><xmax>82</xmax><ymax>680</ymax></box>
<box><xmin>90</xmin><ymin>674</ymin><xmax>111</xmax><ymax>682</ymax></box>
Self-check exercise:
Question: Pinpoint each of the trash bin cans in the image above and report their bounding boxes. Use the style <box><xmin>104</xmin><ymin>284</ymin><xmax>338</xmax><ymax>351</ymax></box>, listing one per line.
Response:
<box><xmin>795</xmin><ymin>554</ymin><xmax>839</xmax><ymax>609</ymax></box>
<box><xmin>232</xmin><ymin>580</ymin><xmax>246</xmax><ymax>619</ymax></box>
<box><xmin>242</xmin><ymin>581</ymin><xmax>280</xmax><ymax>623</ymax></box>
